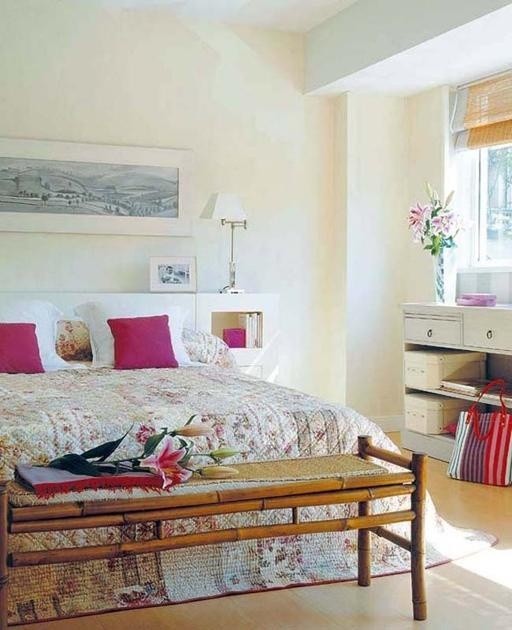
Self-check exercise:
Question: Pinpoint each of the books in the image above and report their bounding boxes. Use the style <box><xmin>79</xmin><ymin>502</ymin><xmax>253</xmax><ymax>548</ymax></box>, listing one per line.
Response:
<box><xmin>239</xmin><ymin>311</ymin><xmax>264</xmax><ymax>349</ymax></box>
<box><xmin>438</xmin><ymin>375</ymin><xmax>512</xmax><ymax>403</ymax></box>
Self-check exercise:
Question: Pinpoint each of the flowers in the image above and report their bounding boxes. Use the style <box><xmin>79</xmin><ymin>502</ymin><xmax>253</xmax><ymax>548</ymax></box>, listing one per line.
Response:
<box><xmin>406</xmin><ymin>179</ymin><xmax>461</xmax><ymax>255</ymax></box>
<box><xmin>11</xmin><ymin>415</ymin><xmax>244</xmax><ymax>495</ymax></box>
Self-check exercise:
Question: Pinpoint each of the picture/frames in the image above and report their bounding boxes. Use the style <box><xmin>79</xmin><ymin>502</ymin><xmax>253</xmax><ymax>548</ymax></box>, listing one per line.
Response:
<box><xmin>0</xmin><ymin>135</ymin><xmax>195</xmax><ymax>239</ymax></box>
<box><xmin>148</xmin><ymin>254</ymin><xmax>197</xmax><ymax>293</ymax></box>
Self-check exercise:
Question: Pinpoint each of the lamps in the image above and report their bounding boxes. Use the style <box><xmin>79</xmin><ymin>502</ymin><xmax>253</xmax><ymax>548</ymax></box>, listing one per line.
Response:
<box><xmin>211</xmin><ymin>190</ymin><xmax>249</xmax><ymax>294</ymax></box>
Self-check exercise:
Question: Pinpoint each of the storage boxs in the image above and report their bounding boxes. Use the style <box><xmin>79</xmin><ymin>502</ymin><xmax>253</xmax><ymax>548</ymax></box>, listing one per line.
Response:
<box><xmin>405</xmin><ymin>348</ymin><xmax>488</xmax><ymax>394</ymax></box>
<box><xmin>403</xmin><ymin>388</ymin><xmax>472</xmax><ymax>433</ymax></box>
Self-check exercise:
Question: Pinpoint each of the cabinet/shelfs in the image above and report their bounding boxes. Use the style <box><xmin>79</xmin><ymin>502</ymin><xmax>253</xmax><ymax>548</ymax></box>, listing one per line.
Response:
<box><xmin>401</xmin><ymin>305</ymin><xmax>511</xmax><ymax>464</ymax></box>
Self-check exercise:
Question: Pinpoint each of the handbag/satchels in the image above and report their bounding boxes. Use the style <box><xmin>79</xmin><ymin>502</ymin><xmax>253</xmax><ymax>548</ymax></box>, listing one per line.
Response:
<box><xmin>447</xmin><ymin>410</ymin><xmax>511</xmax><ymax>486</ymax></box>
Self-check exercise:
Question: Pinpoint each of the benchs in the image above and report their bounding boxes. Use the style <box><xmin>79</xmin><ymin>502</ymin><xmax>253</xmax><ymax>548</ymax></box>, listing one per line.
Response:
<box><xmin>2</xmin><ymin>434</ymin><xmax>433</xmax><ymax>623</ymax></box>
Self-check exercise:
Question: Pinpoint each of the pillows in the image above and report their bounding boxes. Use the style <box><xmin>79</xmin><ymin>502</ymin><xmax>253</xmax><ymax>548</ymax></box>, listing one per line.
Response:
<box><xmin>78</xmin><ymin>301</ymin><xmax>193</xmax><ymax>367</ymax></box>
<box><xmin>105</xmin><ymin>315</ymin><xmax>178</xmax><ymax>370</ymax></box>
<box><xmin>183</xmin><ymin>329</ymin><xmax>242</xmax><ymax>368</ymax></box>
<box><xmin>0</xmin><ymin>297</ymin><xmax>95</xmax><ymax>372</ymax></box>
<box><xmin>1</xmin><ymin>322</ymin><xmax>46</xmax><ymax>375</ymax></box>
<box><xmin>56</xmin><ymin>321</ymin><xmax>94</xmax><ymax>362</ymax></box>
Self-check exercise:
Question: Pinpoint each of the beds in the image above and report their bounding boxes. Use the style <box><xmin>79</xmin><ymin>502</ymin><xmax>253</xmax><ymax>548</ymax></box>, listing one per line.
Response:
<box><xmin>1</xmin><ymin>290</ymin><xmax>448</xmax><ymax>622</ymax></box>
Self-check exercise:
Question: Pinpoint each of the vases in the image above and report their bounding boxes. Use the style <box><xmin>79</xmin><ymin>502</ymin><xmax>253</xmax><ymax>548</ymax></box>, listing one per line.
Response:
<box><xmin>436</xmin><ymin>249</ymin><xmax>446</xmax><ymax>304</ymax></box>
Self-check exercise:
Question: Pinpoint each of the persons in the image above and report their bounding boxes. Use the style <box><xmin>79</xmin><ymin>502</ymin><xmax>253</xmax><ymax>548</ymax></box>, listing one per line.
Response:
<box><xmin>160</xmin><ymin>265</ymin><xmax>183</xmax><ymax>284</ymax></box>
